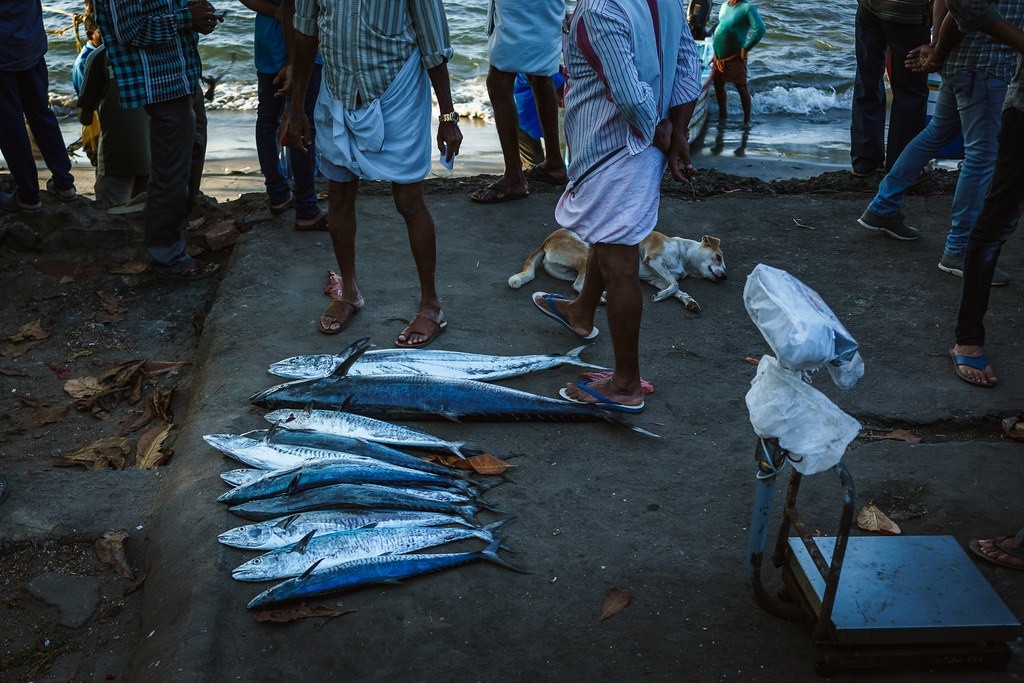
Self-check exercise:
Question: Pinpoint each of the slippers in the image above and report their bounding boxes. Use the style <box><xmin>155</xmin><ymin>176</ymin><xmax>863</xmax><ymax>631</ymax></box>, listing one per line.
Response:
<box><xmin>523</xmin><ymin>163</ymin><xmax>568</xmax><ymax>185</ymax></box>
<box><xmin>46</xmin><ymin>178</ymin><xmax>77</xmax><ymax>202</ymax></box>
<box><xmin>531</xmin><ymin>292</ymin><xmax>600</xmax><ymax>340</ymax></box>
<box><xmin>558</xmin><ymin>380</ymin><xmax>646</xmax><ymax>414</ymax></box>
<box><xmin>0</xmin><ymin>191</ymin><xmax>41</xmax><ymax>216</ymax></box>
<box><xmin>319</xmin><ymin>299</ymin><xmax>361</xmax><ymax>335</ymax></box>
<box><xmin>470</xmin><ymin>183</ymin><xmax>530</xmax><ymax>203</ymax></box>
<box><xmin>395</xmin><ymin>313</ymin><xmax>447</xmax><ymax>349</ymax></box>
<box><xmin>948</xmin><ymin>347</ymin><xmax>998</xmax><ymax>388</ymax></box>
<box><xmin>295</xmin><ymin>211</ymin><xmax>330</xmax><ymax>231</ymax></box>
<box><xmin>270</xmin><ymin>191</ymin><xmax>296</xmax><ymax>214</ymax></box>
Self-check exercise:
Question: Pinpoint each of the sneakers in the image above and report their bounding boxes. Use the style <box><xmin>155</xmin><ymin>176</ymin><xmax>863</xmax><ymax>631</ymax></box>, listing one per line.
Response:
<box><xmin>938</xmin><ymin>254</ymin><xmax>1010</xmax><ymax>285</ymax></box>
<box><xmin>856</xmin><ymin>208</ymin><xmax>919</xmax><ymax>240</ymax></box>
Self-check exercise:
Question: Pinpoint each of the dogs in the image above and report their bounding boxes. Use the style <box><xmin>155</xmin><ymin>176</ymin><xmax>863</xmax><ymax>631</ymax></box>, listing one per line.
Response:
<box><xmin>507</xmin><ymin>228</ymin><xmax>727</xmax><ymax>311</ymax></box>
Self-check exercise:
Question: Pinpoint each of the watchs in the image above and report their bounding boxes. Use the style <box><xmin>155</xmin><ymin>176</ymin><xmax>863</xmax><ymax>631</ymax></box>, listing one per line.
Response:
<box><xmin>439</xmin><ymin>112</ymin><xmax>459</xmax><ymax>124</ymax></box>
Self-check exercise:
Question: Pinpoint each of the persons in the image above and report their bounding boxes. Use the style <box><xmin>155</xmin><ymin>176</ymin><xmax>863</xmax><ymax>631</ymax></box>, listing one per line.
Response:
<box><xmin>73</xmin><ymin>0</ymin><xmax>150</xmax><ymax>199</ymax></box>
<box><xmin>949</xmin><ymin>43</ymin><xmax>1023</xmax><ymax>387</ymax></box>
<box><xmin>277</xmin><ymin>1</ymin><xmax>464</xmax><ymax>346</ymax></box>
<box><xmin>240</xmin><ymin>1</ymin><xmax>330</xmax><ymax>231</ymax></box>
<box><xmin>470</xmin><ymin>0</ymin><xmax>576</xmax><ymax>201</ymax></box>
<box><xmin>0</xmin><ymin>1</ymin><xmax>77</xmax><ymax>213</ymax></box>
<box><xmin>90</xmin><ymin>0</ymin><xmax>224</xmax><ymax>279</ymax></box>
<box><xmin>850</xmin><ymin>1</ymin><xmax>933</xmax><ymax>183</ymax></box>
<box><xmin>708</xmin><ymin>0</ymin><xmax>765</xmax><ymax>123</ymax></box>
<box><xmin>531</xmin><ymin>0</ymin><xmax>702</xmax><ymax>411</ymax></box>
<box><xmin>860</xmin><ymin>0</ymin><xmax>1024</xmax><ymax>287</ymax></box>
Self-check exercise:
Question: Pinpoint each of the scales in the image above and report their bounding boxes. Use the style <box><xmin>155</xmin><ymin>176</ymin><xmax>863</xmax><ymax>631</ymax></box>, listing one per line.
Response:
<box><xmin>741</xmin><ymin>263</ymin><xmax>1024</xmax><ymax>650</ymax></box>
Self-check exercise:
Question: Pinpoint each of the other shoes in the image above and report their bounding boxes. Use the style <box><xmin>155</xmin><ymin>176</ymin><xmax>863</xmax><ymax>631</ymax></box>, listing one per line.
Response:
<box><xmin>286</xmin><ymin>179</ymin><xmax>297</xmax><ymax>193</ymax></box>
<box><xmin>190</xmin><ymin>196</ymin><xmax>236</xmax><ymax>216</ymax></box>
<box><xmin>314</xmin><ymin>177</ymin><xmax>328</xmax><ymax>200</ymax></box>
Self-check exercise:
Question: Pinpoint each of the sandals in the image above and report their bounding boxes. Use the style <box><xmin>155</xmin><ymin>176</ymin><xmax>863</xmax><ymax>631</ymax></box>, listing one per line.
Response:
<box><xmin>1001</xmin><ymin>416</ymin><xmax>1024</xmax><ymax>441</ymax></box>
<box><xmin>850</xmin><ymin>162</ymin><xmax>885</xmax><ymax>177</ymax></box>
<box><xmin>968</xmin><ymin>535</ymin><xmax>1024</xmax><ymax>571</ymax></box>
<box><xmin>910</xmin><ymin>164</ymin><xmax>935</xmax><ymax>186</ymax></box>
<box><xmin>149</xmin><ymin>258</ymin><xmax>221</xmax><ymax>281</ymax></box>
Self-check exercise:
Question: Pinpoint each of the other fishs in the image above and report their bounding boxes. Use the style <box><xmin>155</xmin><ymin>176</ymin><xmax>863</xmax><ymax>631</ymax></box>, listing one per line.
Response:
<box><xmin>216</xmin><ymin>509</ymin><xmax>480</xmax><ymax>550</ymax></box>
<box><xmin>264</xmin><ymin>395</ymin><xmax>467</xmax><ymax>458</ymax></box>
<box><xmin>247</xmin><ymin>535</ymin><xmax>534</xmax><ymax>611</ymax></box>
<box><xmin>243</xmin><ymin>431</ymin><xmax>476</xmax><ymax>484</ymax></box>
<box><xmin>202</xmin><ymin>434</ymin><xmax>478</xmax><ymax>494</ymax></box>
<box><xmin>219</xmin><ymin>468</ymin><xmax>510</xmax><ymax>509</ymax></box>
<box><xmin>231</xmin><ymin>515</ymin><xmax>522</xmax><ymax>583</ymax></box>
<box><xmin>228</xmin><ymin>486</ymin><xmax>482</xmax><ymax>528</ymax></box>
<box><xmin>266</xmin><ymin>345</ymin><xmax>610</xmax><ymax>384</ymax></box>
<box><xmin>249</xmin><ymin>346</ymin><xmax>659</xmax><ymax>436</ymax></box>
<box><xmin>218</xmin><ymin>456</ymin><xmax>474</xmax><ymax>503</ymax></box>
